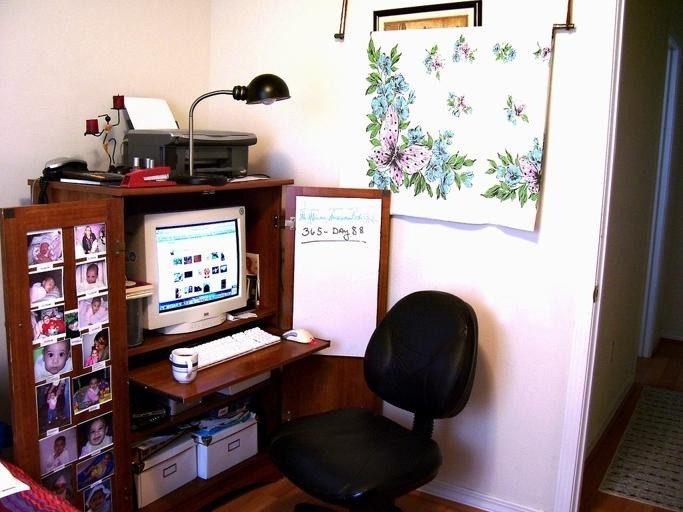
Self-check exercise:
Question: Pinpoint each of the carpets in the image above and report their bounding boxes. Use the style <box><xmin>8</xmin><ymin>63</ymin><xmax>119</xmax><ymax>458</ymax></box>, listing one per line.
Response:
<box><xmin>596</xmin><ymin>385</ymin><xmax>682</xmax><ymax>512</ymax></box>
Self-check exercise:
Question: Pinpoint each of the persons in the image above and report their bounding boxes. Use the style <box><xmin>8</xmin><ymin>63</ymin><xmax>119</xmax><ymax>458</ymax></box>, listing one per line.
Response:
<box><xmin>29</xmin><ymin>223</ymin><xmax>114</xmax><ymax>512</ymax></box>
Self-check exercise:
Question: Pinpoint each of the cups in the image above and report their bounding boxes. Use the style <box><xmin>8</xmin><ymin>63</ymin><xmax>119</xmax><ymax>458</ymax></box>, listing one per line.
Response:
<box><xmin>127</xmin><ymin>299</ymin><xmax>143</xmax><ymax>347</ymax></box>
<box><xmin>169</xmin><ymin>348</ymin><xmax>199</xmax><ymax>384</ymax></box>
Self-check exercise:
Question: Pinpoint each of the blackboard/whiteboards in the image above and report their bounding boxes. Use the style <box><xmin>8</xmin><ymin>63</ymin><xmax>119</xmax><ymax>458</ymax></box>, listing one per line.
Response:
<box><xmin>280</xmin><ymin>184</ymin><xmax>391</xmax><ymax>359</ymax></box>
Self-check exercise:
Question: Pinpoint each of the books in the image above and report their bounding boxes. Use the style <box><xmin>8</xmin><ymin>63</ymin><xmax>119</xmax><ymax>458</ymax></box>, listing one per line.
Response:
<box><xmin>247</xmin><ymin>252</ymin><xmax>261</xmax><ymax>310</ymax></box>
<box><xmin>125</xmin><ymin>279</ymin><xmax>154</xmax><ymax>300</ymax></box>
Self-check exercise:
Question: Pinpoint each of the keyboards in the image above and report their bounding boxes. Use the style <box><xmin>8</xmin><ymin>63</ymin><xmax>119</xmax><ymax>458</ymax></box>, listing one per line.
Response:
<box><xmin>189</xmin><ymin>327</ymin><xmax>281</xmax><ymax>371</ymax></box>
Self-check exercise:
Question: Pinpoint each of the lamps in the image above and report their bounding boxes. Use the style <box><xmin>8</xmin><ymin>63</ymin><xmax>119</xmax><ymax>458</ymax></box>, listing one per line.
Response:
<box><xmin>164</xmin><ymin>74</ymin><xmax>291</xmax><ymax>188</ymax></box>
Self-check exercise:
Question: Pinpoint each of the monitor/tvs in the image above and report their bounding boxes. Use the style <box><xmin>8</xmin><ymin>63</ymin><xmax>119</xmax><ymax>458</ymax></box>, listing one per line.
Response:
<box><xmin>127</xmin><ymin>204</ymin><xmax>249</xmax><ymax>335</ymax></box>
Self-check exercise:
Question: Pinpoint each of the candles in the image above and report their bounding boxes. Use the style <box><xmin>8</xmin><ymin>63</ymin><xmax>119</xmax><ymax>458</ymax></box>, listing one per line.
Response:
<box><xmin>84</xmin><ymin>118</ymin><xmax>99</xmax><ymax>135</ymax></box>
<box><xmin>112</xmin><ymin>94</ymin><xmax>125</xmax><ymax>109</ymax></box>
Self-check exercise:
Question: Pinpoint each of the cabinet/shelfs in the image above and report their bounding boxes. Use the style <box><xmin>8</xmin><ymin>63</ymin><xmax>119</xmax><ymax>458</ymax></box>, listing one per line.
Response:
<box><xmin>0</xmin><ymin>165</ymin><xmax>394</xmax><ymax>511</ymax></box>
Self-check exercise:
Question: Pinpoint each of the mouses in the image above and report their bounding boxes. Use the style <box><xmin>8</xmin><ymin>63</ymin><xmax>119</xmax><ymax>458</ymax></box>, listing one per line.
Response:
<box><xmin>284</xmin><ymin>326</ymin><xmax>314</xmax><ymax>344</ymax></box>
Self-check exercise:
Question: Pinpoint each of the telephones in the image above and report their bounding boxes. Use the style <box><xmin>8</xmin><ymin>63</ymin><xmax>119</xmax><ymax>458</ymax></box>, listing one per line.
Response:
<box><xmin>42</xmin><ymin>156</ymin><xmax>89</xmax><ymax>182</ymax></box>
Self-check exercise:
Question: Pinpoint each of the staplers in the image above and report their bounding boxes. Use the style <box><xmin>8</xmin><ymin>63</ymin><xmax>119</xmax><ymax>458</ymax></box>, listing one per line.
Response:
<box><xmin>120</xmin><ymin>166</ymin><xmax>177</xmax><ymax>188</ymax></box>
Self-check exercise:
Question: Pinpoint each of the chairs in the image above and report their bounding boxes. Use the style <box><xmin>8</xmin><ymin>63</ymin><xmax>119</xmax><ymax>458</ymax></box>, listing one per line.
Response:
<box><xmin>267</xmin><ymin>289</ymin><xmax>479</xmax><ymax>511</ymax></box>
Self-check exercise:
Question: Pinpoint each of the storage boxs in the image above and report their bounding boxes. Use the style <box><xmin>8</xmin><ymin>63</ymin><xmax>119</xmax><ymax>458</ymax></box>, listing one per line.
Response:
<box><xmin>135</xmin><ymin>435</ymin><xmax>196</xmax><ymax>511</ymax></box>
<box><xmin>183</xmin><ymin>410</ymin><xmax>259</xmax><ymax>480</ymax></box>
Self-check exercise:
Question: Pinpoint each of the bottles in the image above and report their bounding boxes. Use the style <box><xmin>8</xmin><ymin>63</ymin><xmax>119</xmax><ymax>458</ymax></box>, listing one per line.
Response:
<box><xmin>132</xmin><ymin>156</ymin><xmax>154</xmax><ymax>171</ymax></box>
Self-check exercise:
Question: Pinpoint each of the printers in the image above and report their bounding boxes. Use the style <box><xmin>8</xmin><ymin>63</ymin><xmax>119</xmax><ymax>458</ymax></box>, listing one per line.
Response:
<box><xmin>121</xmin><ymin>119</ymin><xmax>258</xmax><ymax>179</ymax></box>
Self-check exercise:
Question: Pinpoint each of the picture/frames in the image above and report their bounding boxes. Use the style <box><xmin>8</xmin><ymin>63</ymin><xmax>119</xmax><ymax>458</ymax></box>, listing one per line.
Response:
<box><xmin>370</xmin><ymin>0</ymin><xmax>484</xmax><ymax>35</ymax></box>
